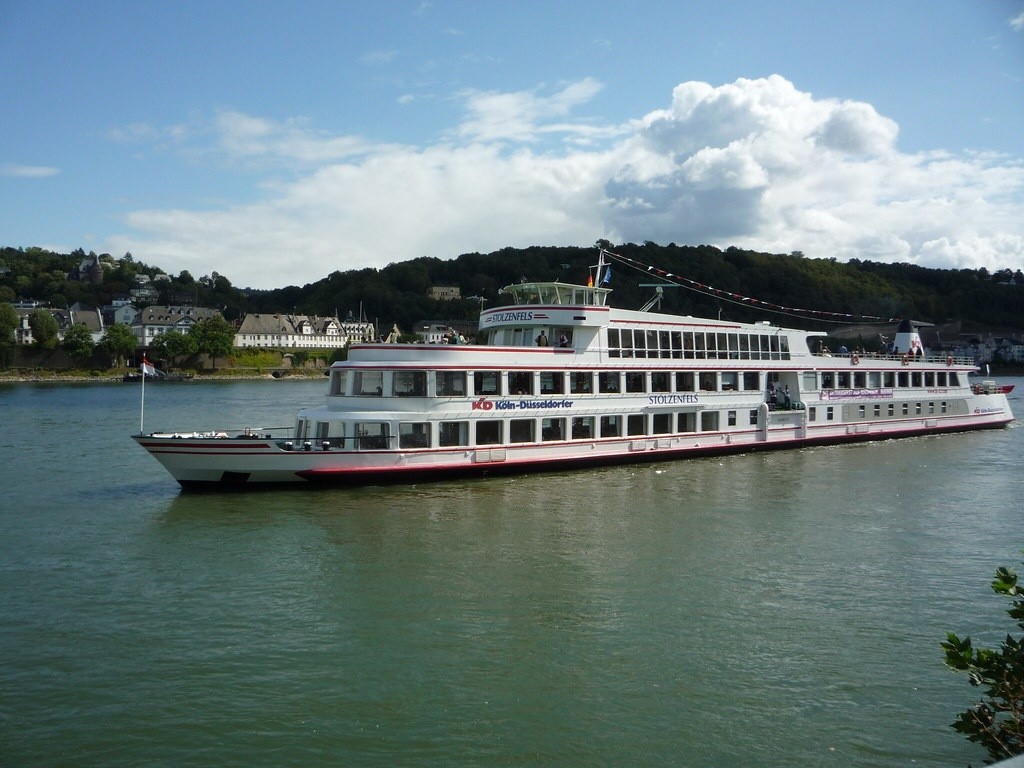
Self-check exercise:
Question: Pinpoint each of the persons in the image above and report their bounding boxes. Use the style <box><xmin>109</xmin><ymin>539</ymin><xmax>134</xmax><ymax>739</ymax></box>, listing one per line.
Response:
<box><xmin>535</xmin><ymin>330</ymin><xmax>548</xmax><ymax>346</ymax></box>
<box><xmin>769</xmin><ymin>386</ymin><xmax>777</xmax><ymax>403</ymax></box>
<box><xmin>555</xmin><ymin>332</ymin><xmax>568</xmax><ymax>347</ymax></box>
<box><xmin>782</xmin><ymin>385</ymin><xmax>790</xmax><ymax>407</ymax></box>
<box><xmin>908</xmin><ymin>346</ymin><xmax>921</xmax><ymax>362</ymax></box>
<box><xmin>840</xmin><ymin>344</ymin><xmax>847</xmax><ymax>357</ymax></box>
<box><xmin>891</xmin><ymin>346</ymin><xmax>898</xmax><ymax>360</ymax></box>
<box><xmin>444</xmin><ymin>330</ymin><xmax>458</xmax><ymax>344</ymax></box>
<box><xmin>818</xmin><ymin>339</ymin><xmax>831</xmax><ymax>356</ymax></box>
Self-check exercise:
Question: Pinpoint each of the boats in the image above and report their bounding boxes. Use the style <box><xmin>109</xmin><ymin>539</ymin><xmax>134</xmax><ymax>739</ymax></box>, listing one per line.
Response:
<box><xmin>969</xmin><ymin>364</ymin><xmax>1014</xmax><ymax>395</ymax></box>
<box><xmin>130</xmin><ymin>246</ymin><xmax>1016</xmax><ymax>489</ymax></box>
<box><xmin>123</xmin><ymin>364</ymin><xmax>193</xmax><ymax>382</ymax></box>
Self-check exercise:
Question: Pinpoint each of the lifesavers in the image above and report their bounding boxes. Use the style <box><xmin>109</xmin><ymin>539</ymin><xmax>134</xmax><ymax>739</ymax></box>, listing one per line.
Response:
<box><xmin>946</xmin><ymin>356</ymin><xmax>953</xmax><ymax>366</ymax></box>
<box><xmin>902</xmin><ymin>355</ymin><xmax>909</xmax><ymax>365</ymax></box>
<box><xmin>851</xmin><ymin>354</ymin><xmax>860</xmax><ymax>364</ymax></box>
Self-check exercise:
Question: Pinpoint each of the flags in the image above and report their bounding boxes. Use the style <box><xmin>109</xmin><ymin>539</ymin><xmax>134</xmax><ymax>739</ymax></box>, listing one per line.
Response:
<box><xmin>143</xmin><ymin>356</ymin><xmax>155</xmax><ymax>375</ymax></box>
<box><xmin>587</xmin><ymin>275</ymin><xmax>593</xmax><ymax>287</ymax></box>
<box><xmin>603</xmin><ymin>266</ymin><xmax>611</xmax><ymax>283</ymax></box>
